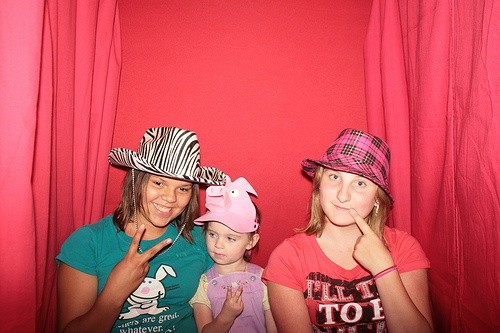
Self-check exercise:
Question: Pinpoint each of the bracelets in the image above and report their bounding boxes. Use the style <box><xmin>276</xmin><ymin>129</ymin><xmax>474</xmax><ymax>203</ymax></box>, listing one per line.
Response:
<box><xmin>372</xmin><ymin>264</ymin><xmax>399</xmax><ymax>282</ymax></box>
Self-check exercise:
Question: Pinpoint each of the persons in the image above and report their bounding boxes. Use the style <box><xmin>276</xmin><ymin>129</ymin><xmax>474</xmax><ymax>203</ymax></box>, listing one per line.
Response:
<box><xmin>260</xmin><ymin>128</ymin><xmax>437</xmax><ymax>333</ymax></box>
<box><xmin>54</xmin><ymin>124</ymin><xmax>216</xmax><ymax>333</ymax></box>
<box><xmin>192</xmin><ymin>183</ymin><xmax>279</xmax><ymax>333</ymax></box>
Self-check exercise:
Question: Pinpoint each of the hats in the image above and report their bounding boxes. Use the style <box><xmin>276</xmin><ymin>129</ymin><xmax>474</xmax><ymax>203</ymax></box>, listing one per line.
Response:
<box><xmin>301</xmin><ymin>127</ymin><xmax>396</xmax><ymax>209</ymax></box>
<box><xmin>109</xmin><ymin>124</ymin><xmax>227</xmax><ymax>190</ymax></box>
<box><xmin>193</xmin><ymin>174</ymin><xmax>258</xmax><ymax>235</ymax></box>
